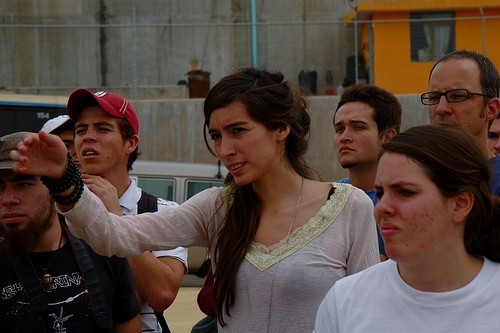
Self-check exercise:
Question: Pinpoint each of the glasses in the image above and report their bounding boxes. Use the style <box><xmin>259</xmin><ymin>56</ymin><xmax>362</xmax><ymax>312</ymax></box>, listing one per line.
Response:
<box><xmin>421</xmin><ymin>88</ymin><xmax>494</xmax><ymax>105</ymax></box>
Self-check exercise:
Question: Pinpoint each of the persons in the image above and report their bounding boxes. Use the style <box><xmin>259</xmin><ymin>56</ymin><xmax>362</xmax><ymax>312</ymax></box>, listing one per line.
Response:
<box><xmin>330</xmin><ymin>84</ymin><xmax>404</xmax><ymax>264</ymax></box>
<box><xmin>421</xmin><ymin>48</ymin><xmax>500</xmax><ymax>196</ymax></box>
<box><xmin>65</xmin><ymin>85</ymin><xmax>191</xmax><ymax>333</ymax></box>
<box><xmin>486</xmin><ymin>114</ymin><xmax>500</xmax><ymax>158</ymax></box>
<box><xmin>0</xmin><ymin>128</ymin><xmax>146</xmax><ymax>332</ymax></box>
<box><xmin>7</xmin><ymin>67</ymin><xmax>382</xmax><ymax>332</ymax></box>
<box><xmin>38</xmin><ymin>112</ymin><xmax>87</xmax><ymax>175</ymax></box>
<box><xmin>310</xmin><ymin>122</ymin><xmax>500</xmax><ymax>332</ymax></box>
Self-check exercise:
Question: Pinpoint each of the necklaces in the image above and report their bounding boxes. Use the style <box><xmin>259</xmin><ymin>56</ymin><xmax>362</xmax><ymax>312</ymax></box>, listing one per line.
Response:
<box><xmin>239</xmin><ymin>173</ymin><xmax>306</xmax><ymax>332</ymax></box>
<box><xmin>13</xmin><ymin>214</ymin><xmax>64</xmax><ymax>284</ymax></box>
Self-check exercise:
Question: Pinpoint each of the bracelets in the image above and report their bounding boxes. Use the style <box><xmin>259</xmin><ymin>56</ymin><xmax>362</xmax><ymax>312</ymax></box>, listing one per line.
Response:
<box><xmin>40</xmin><ymin>152</ymin><xmax>85</xmax><ymax>207</ymax></box>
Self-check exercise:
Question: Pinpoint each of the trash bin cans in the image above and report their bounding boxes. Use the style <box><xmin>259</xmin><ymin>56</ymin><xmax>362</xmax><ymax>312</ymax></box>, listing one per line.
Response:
<box><xmin>188</xmin><ymin>71</ymin><xmax>211</xmax><ymax>99</ymax></box>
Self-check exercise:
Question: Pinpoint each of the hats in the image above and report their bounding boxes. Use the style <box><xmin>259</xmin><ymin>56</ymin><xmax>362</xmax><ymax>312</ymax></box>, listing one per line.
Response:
<box><xmin>0</xmin><ymin>131</ymin><xmax>38</xmax><ymax>169</ymax></box>
<box><xmin>40</xmin><ymin>115</ymin><xmax>74</xmax><ymax>133</ymax></box>
<box><xmin>66</xmin><ymin>87</ymin><xmax>138</xmax><ymax>135</ymax></box>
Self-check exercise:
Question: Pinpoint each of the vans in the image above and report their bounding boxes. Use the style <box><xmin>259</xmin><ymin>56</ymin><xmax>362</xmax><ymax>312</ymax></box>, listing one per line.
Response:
<box><xmin>128</xmin><ymin>158</ymin><xmax>233</xmax><ymax>206</ymax></box>
